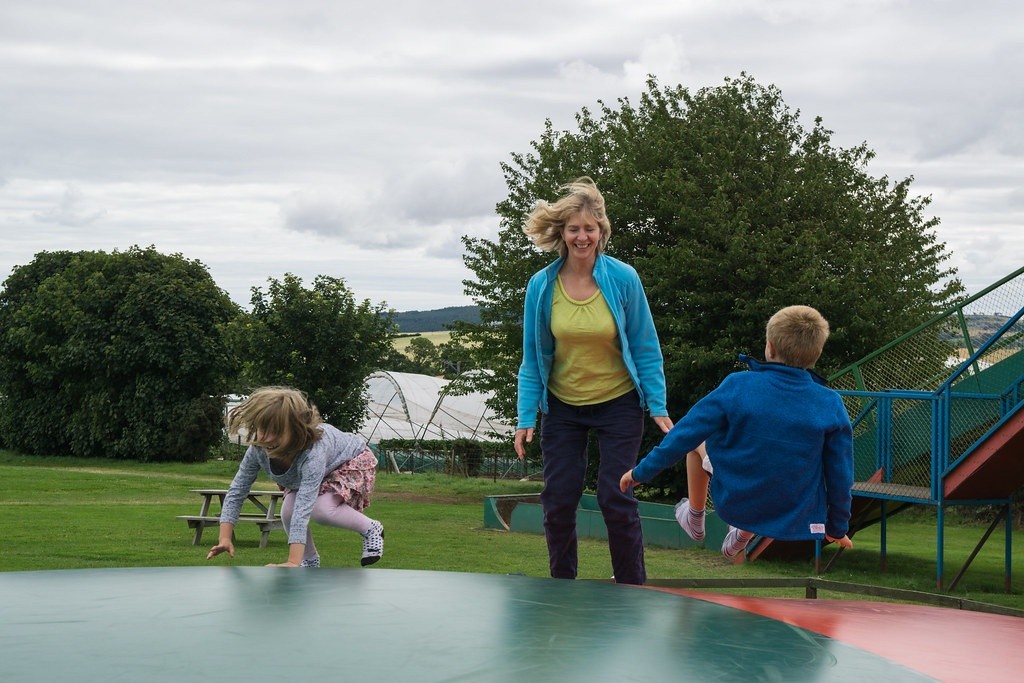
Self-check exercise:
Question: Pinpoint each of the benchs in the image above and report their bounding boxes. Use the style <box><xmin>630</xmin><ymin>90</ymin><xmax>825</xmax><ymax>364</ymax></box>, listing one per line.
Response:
<box><xmin>174</xmin><ymin>512</ymin><xmax>284</xmax><ymax>525</ymax></box>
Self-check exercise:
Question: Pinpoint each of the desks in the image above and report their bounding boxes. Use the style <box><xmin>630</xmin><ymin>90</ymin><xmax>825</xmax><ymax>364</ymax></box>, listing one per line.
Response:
<box><xmin>187</xmin><ymin>489</ymin><xmax>287</xmax><ymax>549</ymax></box>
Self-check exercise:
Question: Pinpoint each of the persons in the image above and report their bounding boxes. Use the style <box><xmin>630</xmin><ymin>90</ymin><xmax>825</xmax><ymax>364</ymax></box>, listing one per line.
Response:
<box><xmin>619</xmin><ymin>305</ymin><xmax>852</xmax><ymax>559</ymax></box>
<box><xmin>206</xmin><ymin>389</ymin><xmax>384</xmax><ymax>567</ymax></box>
<box><xmin>514</xmin><ymin>177</ymin><xmax>675</xmax><ymax>581</ymax></box>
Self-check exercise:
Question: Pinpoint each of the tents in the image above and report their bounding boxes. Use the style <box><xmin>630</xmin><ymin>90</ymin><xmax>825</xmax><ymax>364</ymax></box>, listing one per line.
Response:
<box><xmin>355</xmin><ymin>370</ymin><xmax>515</xmax><ymax>442</ymax></box>
